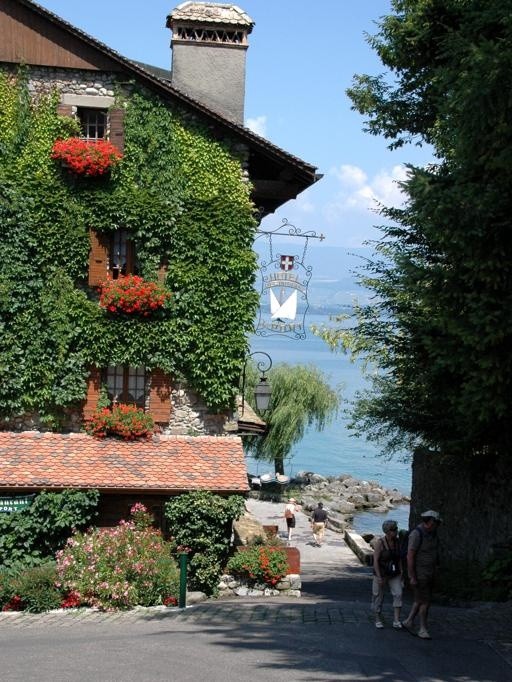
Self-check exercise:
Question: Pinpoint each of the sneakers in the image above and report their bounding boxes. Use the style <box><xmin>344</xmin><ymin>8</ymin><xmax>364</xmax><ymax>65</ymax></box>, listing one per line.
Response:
<box><xmin>392</xmin><ymin>621</ymin><xmax>402</xmax><ymax>628</ymax></box>
<box><xmin>416</xmin><ymin>626</ymin><xmax>432</xmax><ymax>639</ymax></box>
<box><xmin>374</xmin><ymin>620</ymin><xmax>384</xmax><ymax>628</ymax></box>
<box><xmin>402</xmin><ymin>619</ymin><xmax>417</xmax><ymax>636</ymax></box>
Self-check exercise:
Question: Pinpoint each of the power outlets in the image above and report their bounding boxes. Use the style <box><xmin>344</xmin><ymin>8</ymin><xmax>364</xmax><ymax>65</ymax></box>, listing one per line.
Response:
<box><xmin>237</xmin><ymin>350</ymin><xmax>272</xmax><ymax>418</ymax></box>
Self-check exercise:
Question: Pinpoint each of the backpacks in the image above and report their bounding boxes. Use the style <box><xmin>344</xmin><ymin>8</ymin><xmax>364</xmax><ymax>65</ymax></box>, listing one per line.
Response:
<box><xmin>400</xmin><ymin>526</ymin><xmax>424</xmax><ymax>561</ymax></box>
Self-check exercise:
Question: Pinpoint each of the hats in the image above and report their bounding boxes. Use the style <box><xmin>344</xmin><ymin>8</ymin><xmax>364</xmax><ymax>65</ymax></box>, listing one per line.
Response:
<box><xmin>421</xmin><ymin>510</ymin><xmax>442</xmax><ymax>521</ymax></box>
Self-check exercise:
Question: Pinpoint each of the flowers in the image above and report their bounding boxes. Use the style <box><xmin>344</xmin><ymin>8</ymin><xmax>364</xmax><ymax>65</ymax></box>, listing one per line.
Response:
<box><xmin>81</xmin><ymin>402</ymin><xmax>156</xmax><ymax>445</ymax></box>
<box><xmin>50</xmin><ymin>137</ymin><xmax>124</xmax><ymax>177</ymax></box>
<box><xmin>94</xmin><ymin>273</ymin><xmax>171</xmax><ymax>321</ymax></box>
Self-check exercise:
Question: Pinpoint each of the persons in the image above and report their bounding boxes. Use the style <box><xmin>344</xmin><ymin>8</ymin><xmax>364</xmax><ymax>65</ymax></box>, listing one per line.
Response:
<box><xmin>284</xmin><ymin>497</ymin><xmax>299</xmax><ymax>541</ymax></box>
<box><xmin>402</xmin><ymin>509</ymin><xmax>443</xmax><ymax>640</ymax></box>
<box><xmin>372</xmin><ymin>520</ymin><xmax>407</xmax><ymax>629</ymax></box>
<box><xmin>310</xmin><ymin>502</ymin><xmax>329</xmax><ymax>548</ymax></box>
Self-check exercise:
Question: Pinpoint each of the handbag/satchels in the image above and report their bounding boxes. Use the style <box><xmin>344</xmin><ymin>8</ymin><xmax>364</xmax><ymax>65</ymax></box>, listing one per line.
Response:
<box><xmin>371</xmin><ymin>535</ymin><xmax>401</xmax><ymax>579</ymax></box>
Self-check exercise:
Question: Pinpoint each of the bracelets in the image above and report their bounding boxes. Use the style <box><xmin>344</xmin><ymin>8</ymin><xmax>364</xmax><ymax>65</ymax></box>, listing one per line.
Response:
<box><xmin>378</xmin><ymin>575</ymin><xmax>384</xmax><ymax>581</ymax></box>
